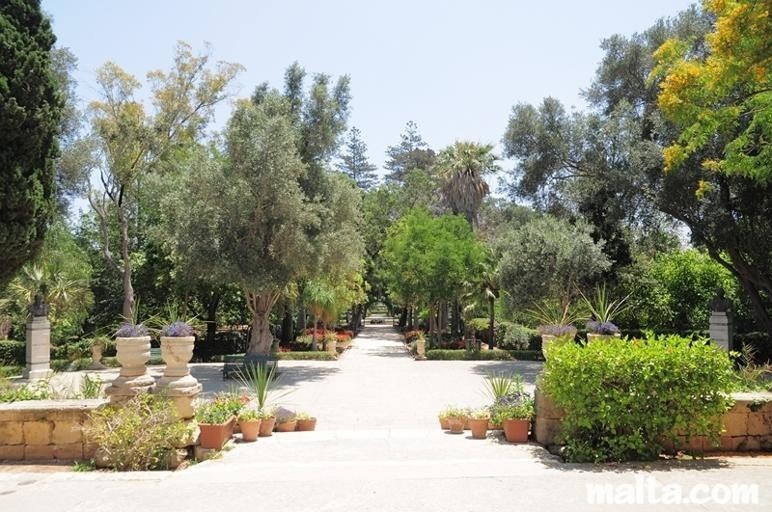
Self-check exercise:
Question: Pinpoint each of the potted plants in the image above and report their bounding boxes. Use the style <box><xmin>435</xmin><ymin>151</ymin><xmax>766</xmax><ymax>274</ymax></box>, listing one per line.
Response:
<box><xmin>439</xmin><ymin>367</ymin><xmax>537</xmax><ymax>442</ymax></box>
<box><xmin>85</xmin><ymin>336</ymin><xmax>108</xmax><ymax>369</ymax></box>
<box><xmin>324</xmin><ymin>328</ymin><xmax>339</xmax><ymax>360</ymax></box>
<box><xmin>197</xmin><ymin>360</ymin><xmax>316</xmax><ymax>450</ymax></box>
<box><xmin>415</xmin><ymin>329</ymin><xmax>428</xmax><ymax>360</ymax></box>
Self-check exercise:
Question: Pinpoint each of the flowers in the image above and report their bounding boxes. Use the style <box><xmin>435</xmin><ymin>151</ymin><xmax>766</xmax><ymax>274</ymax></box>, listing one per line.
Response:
<box><xmin>115</xmin><ymin>324</ymin><xmax>149</xmax><ymax>337</ymax></box>
<box><xmin>537</xmin><ymin>324</ymin><xmax>576</xmax><ymax>337</ymax></box>
<box><xmin>586</xmin><ymin>320</ymin><xmax>619</xmax><ymax>335</ymax></box>
<box><xmin>160</xmin><ymin>321</ymin><xmax>195</xmax><ymax>336</ymax></box>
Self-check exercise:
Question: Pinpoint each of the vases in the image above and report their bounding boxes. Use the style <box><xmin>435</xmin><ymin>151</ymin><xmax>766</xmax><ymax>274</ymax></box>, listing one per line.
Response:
<box><xmin>586</xmin><ymin>333</ymin><xmax>621</xmax><ymax>345</ymax></box>
<box><xmin>160</xmin><ymin>335</ymin><xmax>195</xmax><ymax>377</ymax></box>
<box><xmin>541</xmin><ymin>334</ymin><xmax>574</xmax><ymax>361</ymax></box>
<box><xmin>115</xmin><ymin>336</ymin><xmax>151</xmax><ymax>376</ymax></box>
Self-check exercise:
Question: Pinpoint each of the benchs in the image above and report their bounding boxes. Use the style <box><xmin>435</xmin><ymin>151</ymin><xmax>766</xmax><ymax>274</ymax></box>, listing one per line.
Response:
<box><xmin>223</xmin><ymin>356</ymin><xmax>279</xmax><ymax>380</ymax></box>
